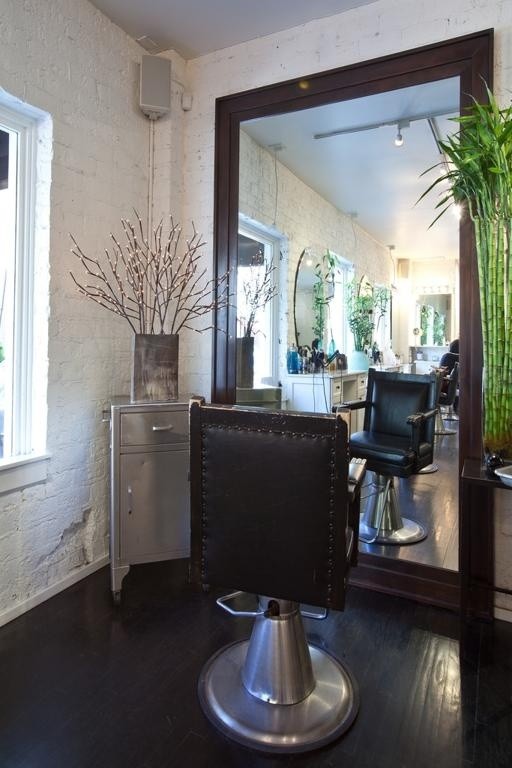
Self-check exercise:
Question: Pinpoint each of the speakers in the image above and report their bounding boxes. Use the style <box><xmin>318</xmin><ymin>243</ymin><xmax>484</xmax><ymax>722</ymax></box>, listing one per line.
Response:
<box><xmin>137</xmin><ymin>53</ymin><xmax>171</xmax><ymax>121</ymax></box>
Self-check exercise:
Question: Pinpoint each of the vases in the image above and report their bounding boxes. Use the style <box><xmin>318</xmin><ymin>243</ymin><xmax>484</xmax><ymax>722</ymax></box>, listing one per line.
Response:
<box><xmin>133</xmin><ymin>334</ymin><xmax>178</xmax><ymax>402</ymax></box>
<box><xmin>237</xmin><ymin>336</ymin><xmax>256</xmax><ymax>386</ymax></box>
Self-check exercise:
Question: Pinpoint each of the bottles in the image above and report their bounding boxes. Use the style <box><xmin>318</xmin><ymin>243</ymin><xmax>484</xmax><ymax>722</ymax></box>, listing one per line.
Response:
<box><xmin>363</xmin><ymin>339</ymin><xmax>396</xmax><ymax>366</ymax></box>
<box><xmin>287</xmin><ymin>338</ymin><xmax>335</xmax><ymax>374</ymax></box>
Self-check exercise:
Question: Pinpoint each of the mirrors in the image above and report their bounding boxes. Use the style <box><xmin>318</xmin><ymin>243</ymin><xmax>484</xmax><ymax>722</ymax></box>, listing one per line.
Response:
<box><xmin>359</xmin><ymin>275</ymin><xmax>373</xmax><ymax>351</ymax></box>
<box><xmin>211</xmin><ymin>29</ymin><xmax>494</xmax><ymax>620</ymax></box>
<box><xmin>295</xmin><ymin>246</ymin><xmax>327</xmax><ymax>354</ymax></box>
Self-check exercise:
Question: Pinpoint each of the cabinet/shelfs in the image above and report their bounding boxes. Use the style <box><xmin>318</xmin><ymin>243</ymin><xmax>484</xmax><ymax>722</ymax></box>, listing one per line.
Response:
<box><xmin>237</xmin><ymin>383</ymin><xmax>282</xmax><ymax>409</ymax></box>
<box><xmin>103</xmin><ymin>403</ymin><xmax>190</xmax><ymax>606</ymax></box>
<box><xmin>373</xmin><ymin>365</ymin><xmax>404</xmax><ymax>374</ymax></box>
<box><xmin>282</xmin><ymin>369</ymin><xmax>369</xmax><ymax>442</ymax></box>
<box><xmin>414</xmin><ymin>361</ymin><xmax>442</xmax><ymax>376</ymax></box>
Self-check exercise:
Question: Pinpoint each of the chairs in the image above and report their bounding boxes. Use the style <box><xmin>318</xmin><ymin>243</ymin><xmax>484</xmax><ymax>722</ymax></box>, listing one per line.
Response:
<box><xmin>332</xmin><ymin>368</ymin><xmax>440</xmax><ymax>547</ymax></box>
<box><xmin>430</xmin><ymin>362</ymin><xmax>458</xmax><ymax>436</ymax></box>
<box><xmin>189</xmin><ymin>395</ymin><xmax>367</xmax><ymax>755</ymax></box>
<box><xmin>440</xmin><ymin>339</ymin><xmax>461</xmax><ymax>422</ymax></box>
